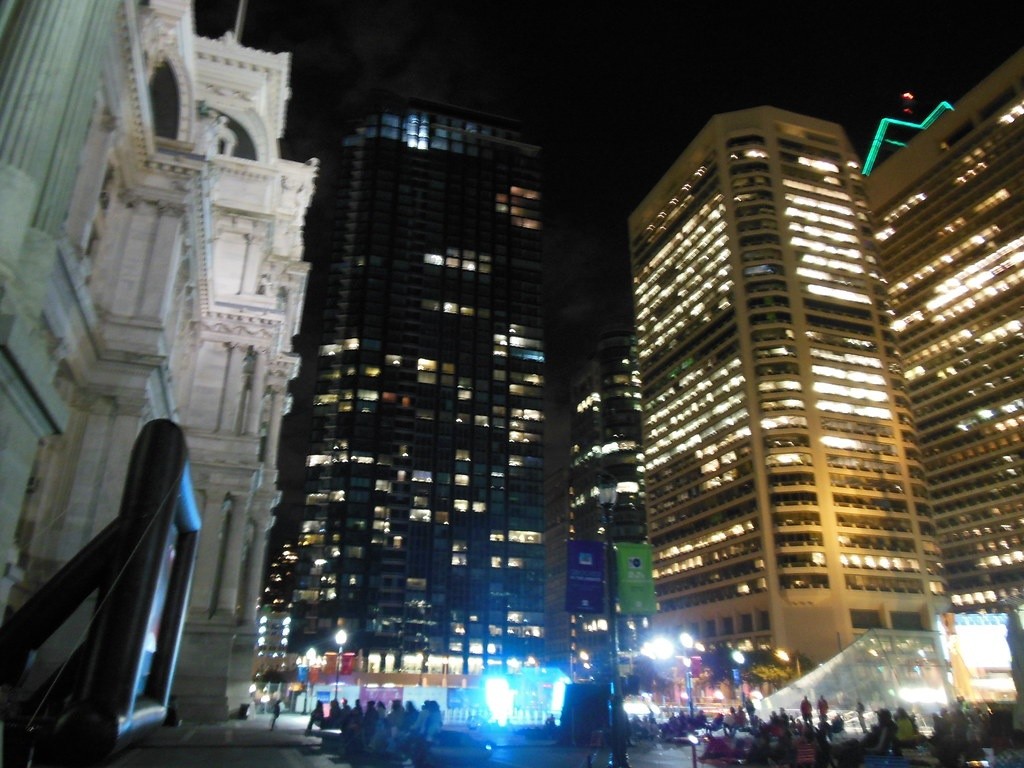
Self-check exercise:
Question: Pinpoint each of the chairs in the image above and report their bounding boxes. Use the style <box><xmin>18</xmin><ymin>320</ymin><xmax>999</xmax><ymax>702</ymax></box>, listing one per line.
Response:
<box><xmin>794</xmin><ymin>743</ymin><xmax>817</xmax><ymax>768</ymax></box>
<box><xmin>863</xmin><ymin>754</ymin><xmax>909</xmax><ymax>768</ymax></box>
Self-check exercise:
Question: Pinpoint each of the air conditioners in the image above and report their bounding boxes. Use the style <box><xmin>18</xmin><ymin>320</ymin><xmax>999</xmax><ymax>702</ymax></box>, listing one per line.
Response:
<box><xmin>100</xmin><ymin>190</ymin><xmax>110</xmax><ymax>209</ymax></box>
<box><xmin>27</xmin><ymin>476</ymin><xmax>40</xmax><ymax>493</ymax></box>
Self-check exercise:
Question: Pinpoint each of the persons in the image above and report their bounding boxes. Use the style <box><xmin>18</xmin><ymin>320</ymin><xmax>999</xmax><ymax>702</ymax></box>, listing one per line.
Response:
<box><xmin>586</xmin><ymin>695</ymin><xmax>1024</xmax><ymax>768</ymax></box>
<box><xmin>467</xmin><ymin>713</ymin><xmax>556</xmax><ymax>738</ymax></box>
<box><xmin>271</xmin><ymin>697</ymin><xmax>283</xmax><ymax>731</ymax></box>
<box><xmin>305</xmin><ymin>698</ymin><xmax>447</xmax><ymax>768</ymax></box>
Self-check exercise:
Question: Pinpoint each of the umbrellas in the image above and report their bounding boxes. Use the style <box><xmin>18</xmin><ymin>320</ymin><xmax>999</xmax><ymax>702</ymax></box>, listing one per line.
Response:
<box><xmin>1006</xmin><ymin>607</ymin><xmax>1024</xmax><ymax>730</ymax></box>
